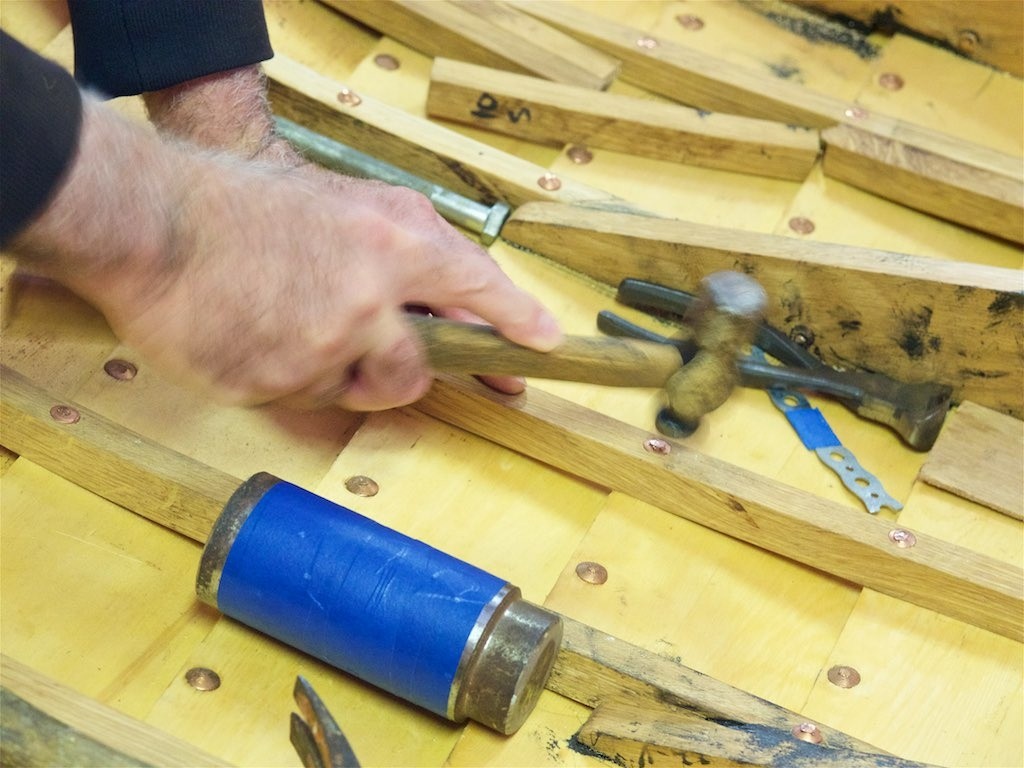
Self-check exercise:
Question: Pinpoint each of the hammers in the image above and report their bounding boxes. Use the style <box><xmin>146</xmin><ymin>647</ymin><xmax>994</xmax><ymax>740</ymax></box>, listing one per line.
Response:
<box><xmin>408</xmin><ymin>271</ymin><xmax>770</xmax><ymax>438</ymax></box>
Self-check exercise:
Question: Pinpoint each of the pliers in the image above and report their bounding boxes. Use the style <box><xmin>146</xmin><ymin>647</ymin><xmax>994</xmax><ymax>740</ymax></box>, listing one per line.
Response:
<box><xmin>599</xmin><ymin>278</ymin><xmax>954</xmax><ymax>452</ymax></box>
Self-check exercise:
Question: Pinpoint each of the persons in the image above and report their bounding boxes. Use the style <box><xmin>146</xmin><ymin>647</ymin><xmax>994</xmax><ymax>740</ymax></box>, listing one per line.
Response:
<box><xmin>0</xmin><ymin>0</ymin><xmax>560</xmax><ymax>411</ymax></box>
